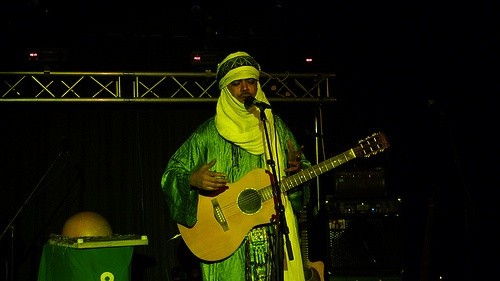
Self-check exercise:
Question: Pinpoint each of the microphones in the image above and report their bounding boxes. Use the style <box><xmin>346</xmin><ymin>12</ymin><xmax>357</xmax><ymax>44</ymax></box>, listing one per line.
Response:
<box><xmin>244</xmin><ymin>96</ymin><xmax>272</xmax><ymax>109</ymax></box>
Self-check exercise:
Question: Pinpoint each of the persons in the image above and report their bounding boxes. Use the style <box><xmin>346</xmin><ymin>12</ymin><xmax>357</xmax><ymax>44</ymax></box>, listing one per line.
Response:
<box><xmin>161</xmin><ymin>51</ymin><xmax>311</xmax><ymax>281</ymax></box>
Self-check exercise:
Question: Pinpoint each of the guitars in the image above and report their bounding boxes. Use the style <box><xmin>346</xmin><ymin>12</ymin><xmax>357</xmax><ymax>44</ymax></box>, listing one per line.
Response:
<box><xmin>297</xmin><ymin>186</ymin><xmax>326</xmax><ymax>281</ymax></box>
<box><xmin>176</xmin><ymin>131</ymin><xmax>390</xmax><ymax>264</ymax></box>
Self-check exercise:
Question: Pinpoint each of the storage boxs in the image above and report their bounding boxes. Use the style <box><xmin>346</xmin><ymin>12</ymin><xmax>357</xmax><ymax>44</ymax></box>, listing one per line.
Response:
<box><xmin>327</xmin><ymin>212</ymin><xmax>406</xmax><ymax>274</ymax></box>
<box><xmin>334</xmin><ymin>170</ymin><xmax>386</xmax><ymax>199</ymax></box>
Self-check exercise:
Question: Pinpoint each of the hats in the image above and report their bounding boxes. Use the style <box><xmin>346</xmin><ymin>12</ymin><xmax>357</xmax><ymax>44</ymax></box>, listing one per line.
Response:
<box><xmin>216</xmin><ymin>52</ymin><xmax>261</xmax><ymax>89</ymax></box>
